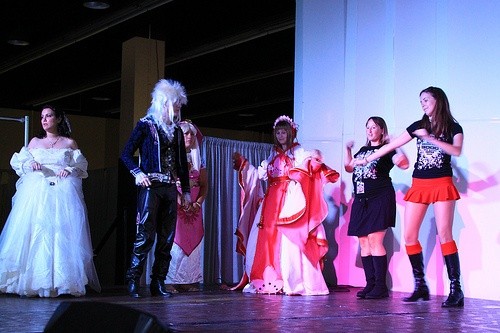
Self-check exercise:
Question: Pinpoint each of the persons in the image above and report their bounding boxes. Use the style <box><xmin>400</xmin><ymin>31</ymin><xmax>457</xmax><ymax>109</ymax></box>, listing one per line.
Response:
<box><xmin>0</xmin><ymin>105</ymin><xmax>104</xmax><ymax>298</ymax></box>
<box><xmin>218</xmin><ymin>115</ymin><xmax>340</xmax><ymax>295</ymax></box>
<box><xmin>144</xmin><ymin>118</ymin><xmax>210</xmax><ymax>294</ymax></box>
<box><xmin>344</xmin><ymin>116</ymin><xmax>410</xmax><ymax>300</ymax></box>
<box><xmin>121</xmin><ymin>79</ymin><xmax>191</xmax><ymax>300</ymax></box>
<box><xmin>354</xmin><ymin>86</ymin><xmax>464</xmax><ymax>308</ymax></box>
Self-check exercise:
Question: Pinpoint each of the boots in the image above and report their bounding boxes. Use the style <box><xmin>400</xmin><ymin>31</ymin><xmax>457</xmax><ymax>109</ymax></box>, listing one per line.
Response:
<box><xmin>403</xmin><ymin>241</ymin><xmax>430</xmax><ymax>302</ymax></box>
<box><xmin>440</xmin><ymin>240</ymin><xmax>464</xmax><ymax>308</ymax></box>
<box><xmin>357</xmin><ymin>255</ymin><xmax>375</xmax><ymax>297</ymax></box>
<box><xmin>364</xmin><ymin>255</ymin><xmax>389</xmax><ymax>298</ymax></box>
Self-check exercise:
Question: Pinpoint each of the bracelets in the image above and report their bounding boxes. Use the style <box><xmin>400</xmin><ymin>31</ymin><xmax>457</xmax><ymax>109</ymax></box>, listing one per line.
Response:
<box><xmin>364</xmin><ymin>157</ymin><xmax>369</xmax><ymax>163</ymax></box>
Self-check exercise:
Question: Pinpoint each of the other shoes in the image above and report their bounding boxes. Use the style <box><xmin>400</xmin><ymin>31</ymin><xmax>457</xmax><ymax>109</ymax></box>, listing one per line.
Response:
<box><xmin>129</xmin><ymin>281</ymin><xmax>141</xmax><ymax>298</ymax></box>
<box><xmin>165</xmin><ymin>289</ymin><xmax>178</xmax><ymax>294</ymax></box>
<box><xmin>187</xmin><ymin>287</ymin><xmax>199</xmax><ymax>291</ymax></box>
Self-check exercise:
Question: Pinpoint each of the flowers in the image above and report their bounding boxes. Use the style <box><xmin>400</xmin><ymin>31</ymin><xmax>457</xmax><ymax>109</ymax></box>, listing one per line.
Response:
<box><xmin>272</xmin><ymin>115</ymin><xmax>301</xmax><ymax>131</ymax></box>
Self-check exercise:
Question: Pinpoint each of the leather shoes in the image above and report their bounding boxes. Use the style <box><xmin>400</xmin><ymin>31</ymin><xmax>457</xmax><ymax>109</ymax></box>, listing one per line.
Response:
<box><xmin>150</xmin><ymin>280</ymin><xmax>174</xmax><ymax>297</ymax></box>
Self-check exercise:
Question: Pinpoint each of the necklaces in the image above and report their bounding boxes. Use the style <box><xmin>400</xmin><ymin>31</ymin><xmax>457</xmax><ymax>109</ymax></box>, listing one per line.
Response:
<box><xmin>47</xmin><ymin>135</ymin><xmax>60</xmax><ymax>148</ymax></box>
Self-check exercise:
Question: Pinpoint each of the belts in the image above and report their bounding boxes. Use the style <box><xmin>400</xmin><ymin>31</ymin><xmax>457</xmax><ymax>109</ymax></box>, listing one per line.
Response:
<box><xmin>149</xmin><ymin>173</ymin><xmax>176</xmax><ymax>184</ymax></box>
